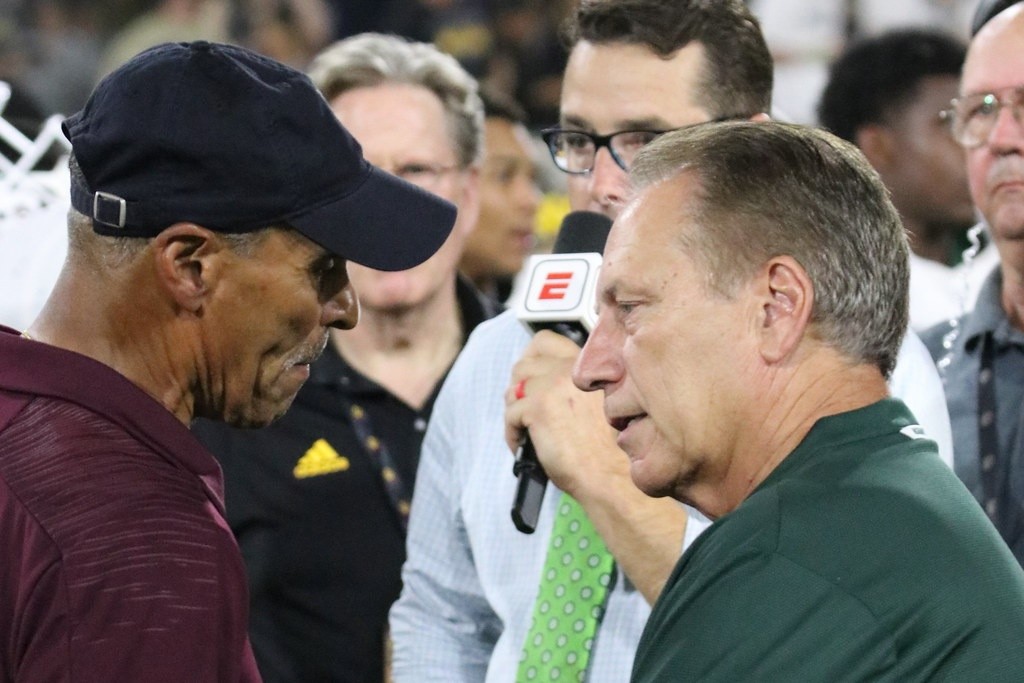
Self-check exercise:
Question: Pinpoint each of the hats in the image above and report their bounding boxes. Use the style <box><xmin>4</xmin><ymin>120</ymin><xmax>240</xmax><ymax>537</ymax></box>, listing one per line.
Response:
<box><xmin>61</xmin><ymin>41</ymin><xmax>458</xmax><ymax>271</ymax></box>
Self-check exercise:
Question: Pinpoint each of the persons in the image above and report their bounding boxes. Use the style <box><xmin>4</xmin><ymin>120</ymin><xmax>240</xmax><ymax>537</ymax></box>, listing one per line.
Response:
<box><xmin>0</xmin><ymin>0</ymin><xmax>1024</xmax><ymax>683</ymax></box>
<box><xmin>0</xmin><ymin>42</ymin><xmax>458</xmax><ymax>683</ymax></box>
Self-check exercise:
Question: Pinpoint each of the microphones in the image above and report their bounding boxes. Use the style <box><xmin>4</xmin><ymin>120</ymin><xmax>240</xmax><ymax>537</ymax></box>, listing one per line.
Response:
<box><xmin>511</xmin><ymin>212</ymin><xmax>612</xmax><ymax>533</ymax></box>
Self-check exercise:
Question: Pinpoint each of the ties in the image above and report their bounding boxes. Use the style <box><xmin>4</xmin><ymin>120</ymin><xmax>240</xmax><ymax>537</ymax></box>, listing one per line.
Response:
<box><xmin>516</xmin><ymin>491</ymin><xmax>615</xmax><ymax>683</ymax></box>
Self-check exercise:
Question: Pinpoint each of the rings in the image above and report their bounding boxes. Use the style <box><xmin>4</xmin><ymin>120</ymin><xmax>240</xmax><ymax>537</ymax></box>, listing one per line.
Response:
<box><xmin>514</xmin><ymin>380</ymin><xmax>525</xmax><ymax>399</ymax></box>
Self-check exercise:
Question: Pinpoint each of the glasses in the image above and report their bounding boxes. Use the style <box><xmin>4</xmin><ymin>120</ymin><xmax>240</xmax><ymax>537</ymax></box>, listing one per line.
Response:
<box><xmin>938</xmin><ymin>87</ymin><xmax>1024</xmax><ymax>149</ymax></box>
<box><xmin>394</xmin><ymin>159</ymin><xmax>456</xmax><ymax>191</ymax></box>
<box><xmin>541</xmin><ymin>114</ymin><xmax>752</xmax><ymax>174</ymax></box>
